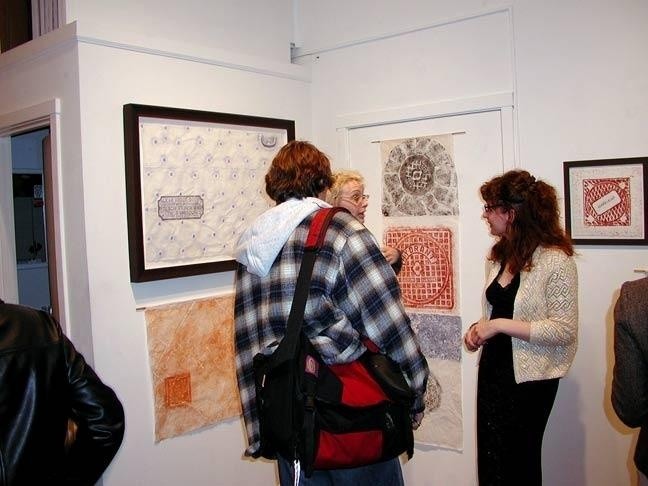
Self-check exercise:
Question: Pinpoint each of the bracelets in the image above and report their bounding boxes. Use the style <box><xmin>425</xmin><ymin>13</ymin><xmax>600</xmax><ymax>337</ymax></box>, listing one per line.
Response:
<box><xmin>411</xmin><ymin>417</ymin><xmax>422</xmax><ymax>428</ymax></box>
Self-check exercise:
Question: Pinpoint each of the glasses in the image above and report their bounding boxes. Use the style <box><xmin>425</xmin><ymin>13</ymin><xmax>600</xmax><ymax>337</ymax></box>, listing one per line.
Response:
<box><xmin>341</xmin><ymin>194</ymin><xmax>369</xmax><ymax>203</ymax></box>
<box><xmin>484</xmin><ymin>203</ymin><xmax>503</xmax><ymax>212</ymax></box>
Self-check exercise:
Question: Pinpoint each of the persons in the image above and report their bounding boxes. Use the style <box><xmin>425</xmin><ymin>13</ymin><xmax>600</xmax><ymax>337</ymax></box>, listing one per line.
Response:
<box><xmin>231</xmin><ymin>139</ymin><xmax>429</xmax><ymax>486</ymax></box>
<box><xmin>0</xmin><ymin>300</ymin><xmax>125</xmax><ymax>486</ymax></box>
<box><xmin>324</xmin><ymin>167</ymin><xmax>402</xmax><ymax>276</ymax></box>
<box><xmin>461</xmin><ymin>167</ymin><xmax>580</xmax><ymax>486</ymax></box>
<box><xmin>610</xmin><ymin>277</ymin><xmax>646</xmax><ymax>485</ymax></box>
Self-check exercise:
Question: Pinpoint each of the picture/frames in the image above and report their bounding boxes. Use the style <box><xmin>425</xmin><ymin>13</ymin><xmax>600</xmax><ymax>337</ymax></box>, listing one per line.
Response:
<box><xmin>562</xmin><ymin>155</ymin><xmax>648</xmax><ymax>248</ymax></box>
<box><xmin>123</xmin><ymin>101</ymin><xmax>297</xmax><ymax>286</ymax></box>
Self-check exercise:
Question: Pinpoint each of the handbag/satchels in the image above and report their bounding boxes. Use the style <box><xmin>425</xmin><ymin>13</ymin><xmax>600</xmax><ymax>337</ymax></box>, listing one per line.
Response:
<box><xmin>255</xmin><ymin>338</ymin><xmax>414</xmax><ymax>468</ymax></box>
